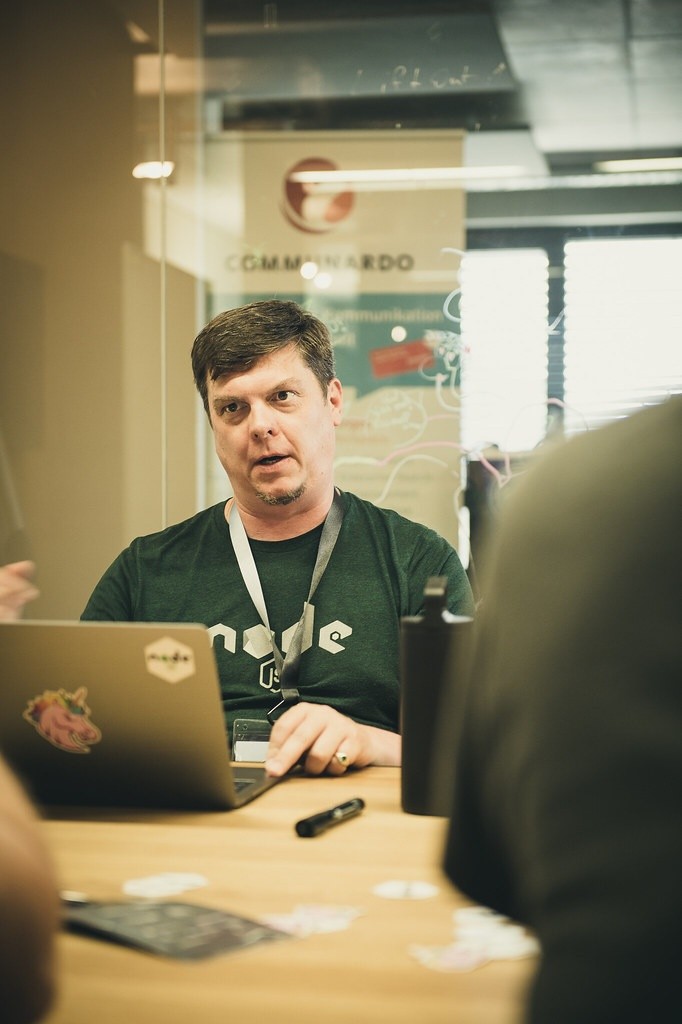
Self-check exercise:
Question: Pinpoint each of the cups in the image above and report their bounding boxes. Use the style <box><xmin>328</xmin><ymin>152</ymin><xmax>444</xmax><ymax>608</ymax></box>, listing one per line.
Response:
<box><xmin>401</xmin><ymin>580</ymin><xmax>473</xmax><ymax>818</ymax></box>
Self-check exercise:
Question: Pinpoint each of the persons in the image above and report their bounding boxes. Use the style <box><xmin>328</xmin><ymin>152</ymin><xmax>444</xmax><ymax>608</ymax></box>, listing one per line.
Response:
<box><xmin>437</xmin><ymin>393</ymin><xmax>682</xmax><ymax>1022</ymax></box>
<box><xmin>1</xmin><ymin>754</ymin><xmax>60</xmax><ymax>1024</ymax></box>
<box><xmin>0</xmin><ymin>299</ymin><xmax>476</xmax><ymax>780</ymax></box>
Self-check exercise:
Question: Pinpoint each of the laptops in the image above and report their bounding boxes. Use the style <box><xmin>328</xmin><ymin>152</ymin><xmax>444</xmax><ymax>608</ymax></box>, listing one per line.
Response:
<box><xmin>0</xmin><ymin>620</ymin><xmax>300</xmax><ymax>812</ymax></box>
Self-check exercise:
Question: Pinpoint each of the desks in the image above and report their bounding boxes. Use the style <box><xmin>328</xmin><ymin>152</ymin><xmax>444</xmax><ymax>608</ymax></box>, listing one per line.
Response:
<box><xmin>31</xmin><ymin>759</ymin><xmax>547</xmax><ymax>1024</ymax></box>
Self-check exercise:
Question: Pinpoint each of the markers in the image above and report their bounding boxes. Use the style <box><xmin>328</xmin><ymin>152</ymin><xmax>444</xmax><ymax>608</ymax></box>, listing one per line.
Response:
<box><xmin>296</xmin><ymin>798</ymin><xmax>365</xmax><ymax>838</ymax></box>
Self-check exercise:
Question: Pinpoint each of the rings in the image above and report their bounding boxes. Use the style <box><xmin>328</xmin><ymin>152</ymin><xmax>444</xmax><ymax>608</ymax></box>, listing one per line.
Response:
<box><xmin>336</xmin><ymin>752</ymin><xmax>351</xmax><ymax>767</ymax></box>
